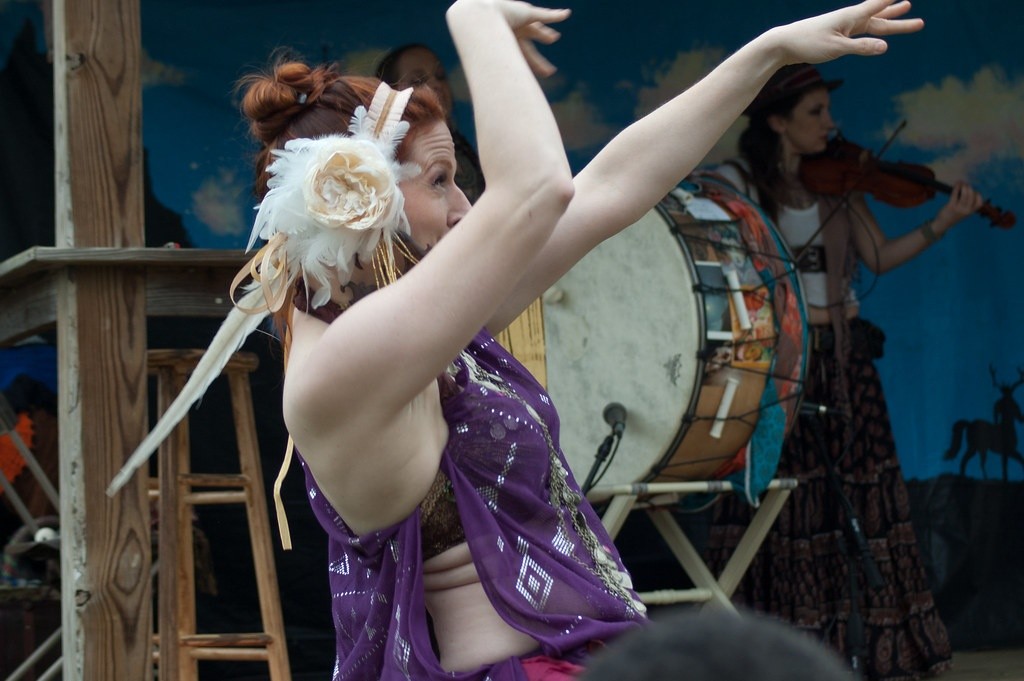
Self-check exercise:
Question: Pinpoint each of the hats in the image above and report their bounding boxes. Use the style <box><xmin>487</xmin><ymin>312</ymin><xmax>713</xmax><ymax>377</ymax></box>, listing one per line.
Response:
<box><xmin>742</xmin><ymin>63</ymin><xmax>844</xmax><ymax>119</ymax></box>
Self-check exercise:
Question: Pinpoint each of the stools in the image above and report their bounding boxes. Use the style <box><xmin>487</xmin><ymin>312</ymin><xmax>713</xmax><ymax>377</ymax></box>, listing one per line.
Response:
<box><xmin>144</xmin><ymin>348</ymin><xmax>296</xmax><ymax>681</ymax></box>
<box><xmin>588</xmin><ymin>477</ymin><xmax>797</xmax><ymax>619</ymax></box>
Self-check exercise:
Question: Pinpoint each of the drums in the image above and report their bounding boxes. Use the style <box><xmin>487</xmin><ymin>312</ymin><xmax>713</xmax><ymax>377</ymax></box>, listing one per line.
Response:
<box><xmin>544</xmin><ymin>175</ymin><xmax>810</xmax><ymax>508</ymax></box>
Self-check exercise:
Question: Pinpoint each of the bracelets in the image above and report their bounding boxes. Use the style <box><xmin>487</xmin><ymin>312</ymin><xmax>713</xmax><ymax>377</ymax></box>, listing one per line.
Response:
<box><xmin>922</xmin><ymin>219</ymin><xmax>943</xmax><ymax>241</ymax></box>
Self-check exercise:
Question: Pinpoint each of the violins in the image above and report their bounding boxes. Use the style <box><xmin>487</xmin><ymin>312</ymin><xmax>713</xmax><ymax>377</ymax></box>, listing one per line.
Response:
<box><xmin>800</xmin><ymin>134</ymin><xmax>1019</xmax><ymax>228</ymax></box>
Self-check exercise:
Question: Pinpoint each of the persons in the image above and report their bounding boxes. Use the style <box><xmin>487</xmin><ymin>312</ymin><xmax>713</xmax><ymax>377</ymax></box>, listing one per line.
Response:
<box><xmin>376</xmin><ymin>44</ymin><xmax>485</xmax><ymax>206</ymax></box>
<box><xmin>711</xmin><ymin>63</ymin><xmax>986</xmax><ymax>681</ymax></box>
<box><xmin>240</xmin><ymin>0</ymin><xmax>926</xmax><ymax>680</ymax></box>
<box><xmin>580</xmin><ymin>604</ymin><xmax>854</xmax><ymax>681</ymax></box>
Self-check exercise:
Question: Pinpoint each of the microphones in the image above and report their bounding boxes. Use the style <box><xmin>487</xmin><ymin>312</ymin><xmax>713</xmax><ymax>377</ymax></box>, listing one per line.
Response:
<box><xmin>603</xmin><ymin>403</ymin><xmax>627</xmax><ymax>439</ymax></box>
<box><xmin>798</xmin><ymin>401</ymin><xmax>839</xmax><ymax>416</ymax></box>
